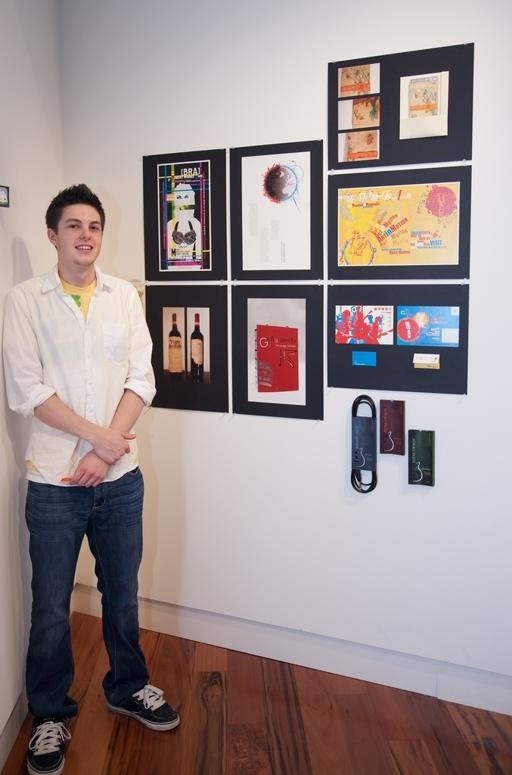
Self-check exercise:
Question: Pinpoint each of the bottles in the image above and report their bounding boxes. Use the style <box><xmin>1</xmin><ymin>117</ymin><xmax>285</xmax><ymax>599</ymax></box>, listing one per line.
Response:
<box><xmin>168</xmin><ymin>313</ymin><xmax>204</xmax><ymax>382</ymax></box>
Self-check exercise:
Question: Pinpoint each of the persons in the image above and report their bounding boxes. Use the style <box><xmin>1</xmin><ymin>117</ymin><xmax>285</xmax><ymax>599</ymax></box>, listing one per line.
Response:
<box><xmin>1</xmin><ymin>183</ymin><xmax>180</xmax><ymax>775</ymax></box>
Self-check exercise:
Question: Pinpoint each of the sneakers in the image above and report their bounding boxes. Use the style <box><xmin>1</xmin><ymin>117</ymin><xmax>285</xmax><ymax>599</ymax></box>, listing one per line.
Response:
<box><xmin>26</xmin><ymin>716</ymin><xmax>72</xmax><ymax>775</ymax></box>
<box><xmin>105</xmin><ymin>682</ymin><xmax>181</xmax><ymax>731</ymax></box>
